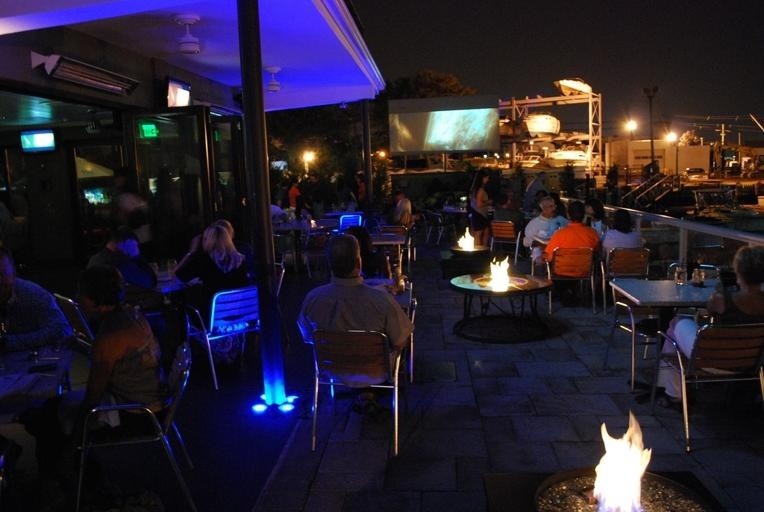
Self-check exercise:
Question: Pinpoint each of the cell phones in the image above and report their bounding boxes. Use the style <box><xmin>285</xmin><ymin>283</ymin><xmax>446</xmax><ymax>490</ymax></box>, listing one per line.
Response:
<box><xmin>29</xmin><ymin>362</ymin><xmax>57</xmax><ymax>373</ymax></box>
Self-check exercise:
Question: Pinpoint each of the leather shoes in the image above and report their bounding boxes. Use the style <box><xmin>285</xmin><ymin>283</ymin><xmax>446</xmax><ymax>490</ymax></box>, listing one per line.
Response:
<box><xmin>656</xmin><ymin>400</ymin><xmax>681</xmax><ymax>410</ymax></box>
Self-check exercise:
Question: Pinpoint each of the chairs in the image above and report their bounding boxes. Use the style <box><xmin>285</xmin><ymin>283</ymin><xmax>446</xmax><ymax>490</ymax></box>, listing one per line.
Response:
<box><xmin>655</xmin><ymin>323</ymin><xmax>764</xmax><ymax>452</ymax></box>
<box><xmin>73</xmin><ymin>337</ymin><xmax>199</xmax><ymax>510</ymax></box>
<box><xmin>310</xmin><ymin>328</ymin><xmax>405</xmax><ymax>459</ymax></box>
<box><xmin>52</xmin><ymin>187</ymin><xmax>653</xmax><ymax>390</ymax></box>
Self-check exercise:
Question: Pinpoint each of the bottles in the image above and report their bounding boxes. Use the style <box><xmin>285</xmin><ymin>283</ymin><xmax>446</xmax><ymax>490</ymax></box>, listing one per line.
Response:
<box><xmin>674</xmin><ymin>263</ymin><xmax>705</xmax><ymax>288</ymax></box>
<box><xmin>32</xmin><ymin>345</ymin><xmax>40</xmax><ymax>364</ymax></box>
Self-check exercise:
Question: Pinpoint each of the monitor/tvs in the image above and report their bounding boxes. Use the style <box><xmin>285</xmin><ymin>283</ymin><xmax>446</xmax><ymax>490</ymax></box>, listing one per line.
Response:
<box><xmin>271</xmin><ymin>159</ymin><xmax>289</xmax><ymax>171</ymax></box>
<box><xmin>17</xmin><ymin>128</ymin><xmax>58</xmax><ymax>153</ymax></box>
<box><xmin>159</xmin><ymin>74</ymin><xmax>194</xmax><ymax>113</ymax></box>
<box><xmin>80</xmin><ymin>184</ymin><xmax>113</xmax><ymax>209</ymax></box>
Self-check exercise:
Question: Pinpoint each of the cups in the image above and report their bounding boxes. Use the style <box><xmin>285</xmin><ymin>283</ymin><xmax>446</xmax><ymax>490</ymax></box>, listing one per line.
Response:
<box><xmin>147</xmin><ymin>257</ymin><xmax>178</xmax><ymax>280</ymax></box>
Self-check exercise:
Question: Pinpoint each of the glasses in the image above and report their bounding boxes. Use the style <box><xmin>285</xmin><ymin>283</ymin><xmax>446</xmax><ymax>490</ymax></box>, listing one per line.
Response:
<box><xmin>546</xmin><ymin>205</ymin><xmax>557</xmax><ymax>208</ymax></box>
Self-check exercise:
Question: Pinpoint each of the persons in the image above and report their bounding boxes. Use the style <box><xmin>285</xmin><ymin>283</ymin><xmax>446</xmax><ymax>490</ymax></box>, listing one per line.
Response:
<box><xmin>272</xmin><ymin>170</ymin><xmax>367</xmax><ymax>250</ymax></box>
<box><xmin>549</xmin><ymin>192</ymin><xmax>566</xmax><ymax>218</ymax></box>
<box><xmin>493</xmin><ymin>194</ymin><xmax>527</xmax><ymax>259</ymax></box>
<box><xmin>580</xmin><ymin>199</ymin><xmax>607</xmax><ymax>234</ymax></box>
<box><xmin>530</xmin><ymin>189</ymin><xmax>548</xmax><ymax>213</ymax></box>
<box><xmin>523</xmin><ymin>172</ymin><xmax>549</xmax><ymax>210</ymax></box>
<box><xmin>544</xmin><ymin>201</ymin><xmax>599</xmax><ymax>303</ymax></box>
<box><xmin>1</xmin><ymin>254</ymin><xmax>72</xmax><ymax>479</ymax></box>
<box><xmin>522</xmin><ymin>196</ymin><xmax>568</xmax><ymax>275</ymax></box>
<box><xmin>393</xmin><ymin>190</ymin><xmax>412</xmax><ymax>226</ymax></box>
<box><xmin>468</xmin><ymin>170</ymin><xmax>494</xmax><ymax>245</ymax></box>
<box><xmin>602</xmin><ymin>209</ymin><xmax>641</xmax><ymax>274</ymax></box>
<box><xmin>85</xmin><ymin>229</ymin><xmax>129</xmax><ymax>272</ymax></box>
<box><xmin>656</xmin><ymin>245</ymin><xmax>764</xmax><ymax>414</ymax></box>
<box><xmin>115</xmin><ymin>168</ymin><xmax>155</xmax><ymax>244</ymax></box>
<box><xmin>564</xmin><ymin>166</ymin><xmax>575</xmax><ymax>197</ymax></box>
<box><xmin>297</xmin><ymin>234</ymin><xmax>414</xmax><ymax>415</ymax></box>
<box><xmin>606</xmin><ymin>166</ymin><xmax>618</xmax><ymax>193</ymax></box>
<box><xmin>60</xmin><ymin>264</ymin><xmax>172</xmax><ymax>510</ymax></box>
<box><xmin>511</xmin><ymin>166</ymin><xmax>527</xmax><ymax>199</ymax></box>
<box><xmin>161</xmin><ymin>221</ymin><xmax>247</xmax><ymax>363</ymax></box>
